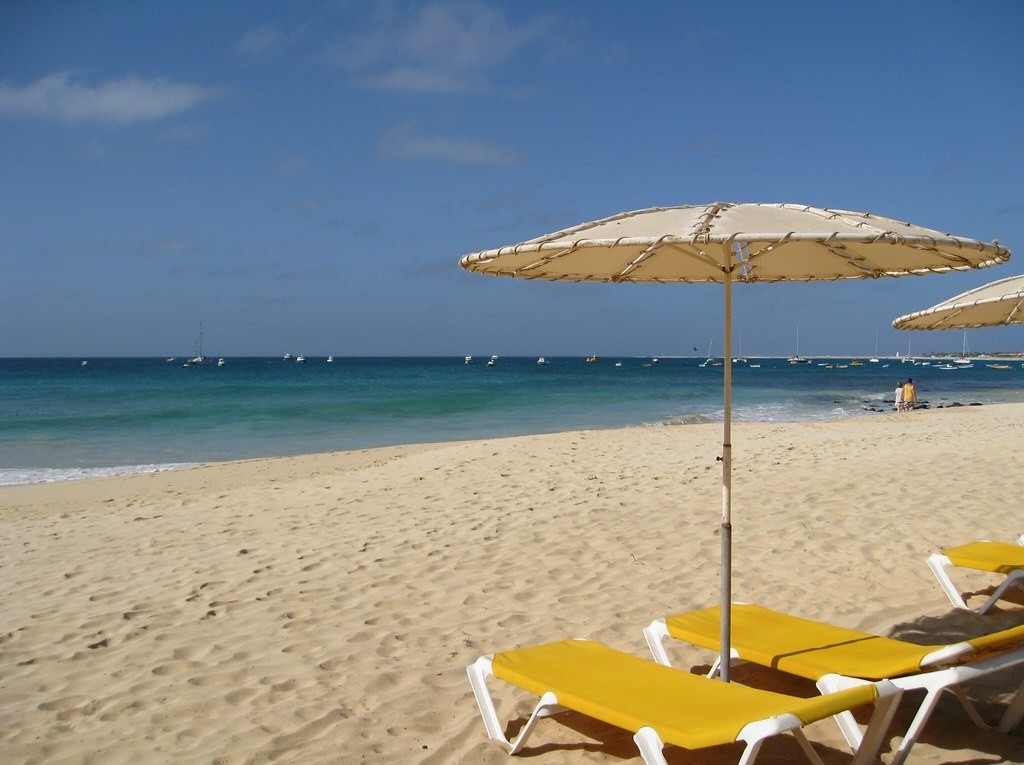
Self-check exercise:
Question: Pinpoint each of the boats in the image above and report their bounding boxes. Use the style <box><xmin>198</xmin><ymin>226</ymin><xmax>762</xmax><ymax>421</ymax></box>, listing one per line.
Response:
<box><xmin>326</xmin><ymin>355</ymin><xmax>334</xmax><ymax>362</ymax></box>
<box><xmin>488</xmin><ymin>359</ymin><xmax>496</xmax><ymax>366</ymax></box>
<box><xmin>932</xmin><ymin>365</ymin><xmax>946</xmax><ymax>368</ymax></box>
<box><xmin>836</xmin><ymin>364</ymin><xmax>848</xmax><ymax>368</ymax></box>
<box><xmin>991</xmin><ymin>365</ymin><xmax>1014</xmax><ymax>370</ymax></box>
<box><xmin>882</xmin><ymin>364</ymin><xmax>890</xmax><ymax>368</ymax></box>
<box><xmin>825</xmin><ymin>365</ymin><xmax>833</xmax><ymax>369</ymax></box>
<box><xmin>704</xmin><ymin>362</ymin><xmax>708</xmax><ymax>366</ymax></box>
<box><xmin>922</xmin><ymin>362</ymin><xmax>930</xmax><ymax>366</ymax></box>
<box><xmin>940</xmin><ymin>367</ymin><xmax>958</xmax><ymax>370</ymax></box>
<box><xmin>652</xmin><ymin>358</ymin><xmax>658</xmax><ymax>363</ymax></box>
<box><xmin>165</xmin><ymin>358</ymin><xmax>175</xmax><ymax>362</ymax></box>
<box><xmin>586</xmin><ymin>354</ymin><xmax>599</xmax><ymax>362</ymax></box>
<box><xmin>947</xmin><ymin>363</ymin><xmax>955</xmax><ymax>367</ymax></box>
<box><xmin>615</xmin><ymin>363</ymin><xmax>623</xmax><ymax>367</ymax></box>
<box><xmin>297</xmin><ymin>354</ymin><xmax>306</xmax><ymax>363</ymax></box>
<box><xmin>699</xmin><ymin>364</ymin><xmax>706</xmax><ymax>368</ymax></box>
<box><xmin>849</xmin><ymin>358</ymin><xmax>864</xmax><ymax>366</ymax></box>
<box><xmin>712</xmin><ymin>362</ymin><xmax>722</xmax><ymax>366</ymax></box>
<box><xmin>986</xmin><ymin>363</ymin><xmax>998</xmax><ymax>368</ymax></box>
<box><xmin>750</xmin><ymin>365</ymin><xmax>760</xmax><ymax>368</ymax></box>
<box><xmin>914</xmin><ymin>362</ymin><xmax>921</xmax><ymax>366</ymax></box>
<box><xmin>789</xmin><ymin>361</ymin><xmax>797</xmax><ymax>365</ymax></box>
<box><xmin>643</xmin><ymin>363</ymin><xmax>653</xmax><ymax>367</ymax></box>
<box><xmin>81</xmin><ymin>360</ymin><xmax>88</xmax><ymax>367</ymax></box>
<box><xmin>464</xmin><ymin>356</ymin><xmax>473</xmax><ymax>365</ymax></box>
<box><xmin>218</xmin><ymin>358</ymin><xmax>225</xmax><ymax>367</ymax></box>
<box><xmin>283</xmin><ymin>353</ymin><xmax>291</xmax><ymax>360</ymax></box>
<box><xmin>807</xmin><ymin>360</ymin><xmax>813</xmax><ymax>364</ymax></box>
<box><xmin>537</xmin><ymin>357</ymin><xmax>550</xmax><ymax>365</ymax></box>
<box><xmin>182</xmin><ymin>364</ymin><xmax>192</xmax><ymax>368</ymax></box>
<box><xmin>492</xmin><ymin>355</ymin><xmax>499</xmax><ymax>360</ymax></box>
<box><xmin>818</xmin><ymin>363</ymin><xmax>829</xmax><ymax>367</ymax></box>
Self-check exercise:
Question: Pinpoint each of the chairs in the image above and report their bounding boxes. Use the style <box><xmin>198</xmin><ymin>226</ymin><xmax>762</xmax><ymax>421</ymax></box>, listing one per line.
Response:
<box><xmin>467</xmin><ymin>534</ymin><xmax>1024</xmax><ymax>765</ymax></box>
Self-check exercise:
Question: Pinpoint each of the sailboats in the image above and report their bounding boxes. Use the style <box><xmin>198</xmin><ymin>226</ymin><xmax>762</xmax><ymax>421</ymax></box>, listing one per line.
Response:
<box><xmin>186</xmin><ymin>322</ymin><xmax>213</xmax><ymax>365</ymax></box>
<box><xmin>952</xmin><ymin>330</ymin><xmax>976</xmax><ymax>368</ymax></box>
<box><xmin>901</xmin><ymin>338</ymin><xmax>915</xmax><ymax>364</ymax></box>
<box><xmin>706</xmin><ymin>336</ymin><xmax>714</xmax><ymax>363</ymax></box>
<box><xmin>787</xmin><ymin>325</ymin><xmax>808</xmax><ymax>362</ymax></box>
<box><xmin>732</xmin><ymin>334</ymin><xmax>748</xmax><ymax>364</ymax></box>
<box><xmin>870</xmin><ymin>332</ymin><xmax>880</xmax><ymax>363</ymax></box>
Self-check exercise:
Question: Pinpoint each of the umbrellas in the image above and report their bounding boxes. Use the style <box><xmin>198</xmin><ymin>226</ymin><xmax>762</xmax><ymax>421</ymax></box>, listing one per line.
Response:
<box><xmin>892</xmin><ymin>273</ymin><xmax>1024</xmax><ymax>330</ymax></box>
<box><xmin>459</xmin><ymin>202</ymin><xmax>1011</xmax><ymax>683</ymax></box>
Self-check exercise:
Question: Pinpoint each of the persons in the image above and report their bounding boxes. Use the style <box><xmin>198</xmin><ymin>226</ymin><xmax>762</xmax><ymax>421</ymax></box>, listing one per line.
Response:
<box><xmin>902</xmin><ymin>378</ymin><xmax>917</xmax><ymax>412</ymax></box>
<box><xmin>894</xmin><ymin>382</ymin><xmax>904</xmax><ymax>413</ymax></box>
